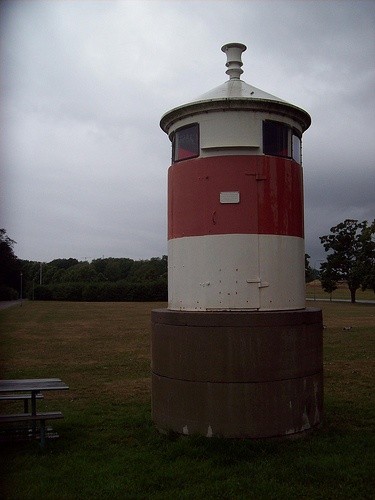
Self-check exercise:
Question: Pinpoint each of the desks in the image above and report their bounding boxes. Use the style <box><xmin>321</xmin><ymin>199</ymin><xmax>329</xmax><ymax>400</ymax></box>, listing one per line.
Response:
<box><xmin>0</xmin><ymin>377</ymin><xmax>69</xmax><ymax>412</ymax></box>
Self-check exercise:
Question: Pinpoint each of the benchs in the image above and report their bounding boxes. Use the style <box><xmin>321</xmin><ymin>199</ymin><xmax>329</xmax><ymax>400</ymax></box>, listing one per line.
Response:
<box><xmin>0</xmin><ymin>393</ymin><xmax>64</xmax><ymax>442</ymax></box>
